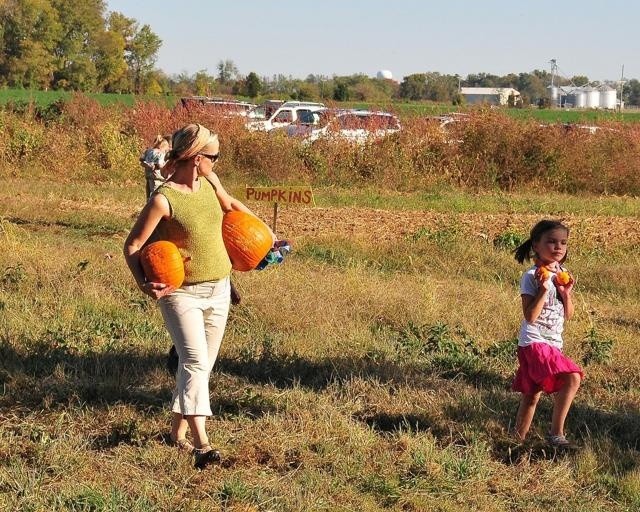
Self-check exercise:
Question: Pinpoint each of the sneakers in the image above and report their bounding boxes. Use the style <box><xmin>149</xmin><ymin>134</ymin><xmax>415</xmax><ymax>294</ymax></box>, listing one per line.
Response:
<box><xmin>550</xmin><ymin>436</ymin><xmax>577</xmax><ymax>449</ymax></box>
<box><xmin>168</xmin><ymin>435</ymin><xmax>193</xmax><ymax>452</ymax></box>
<box><xmin>195</xmin><ymin>449</ymin><xmax>220</xmax><ymax>467</ymax></box>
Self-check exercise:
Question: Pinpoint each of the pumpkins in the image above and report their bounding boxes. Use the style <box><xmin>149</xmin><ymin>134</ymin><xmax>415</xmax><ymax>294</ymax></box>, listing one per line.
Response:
<box><xmin>536</xmin><ymin>266</ymin><xmax>548</xmax><ymax>281</ymax></box>
<box><xmin>221</xmin><ymin>211</ymin><xmax>274</xmax><ymax>271</ymax></box>
<box><xmin>557</xmin><ymin>272</ymin><xmax>570</xmax><ymax>286</ymax></box>
<box><xmin>138</xmin><ymin>241</ymin><xmax>192</xmax><ymax>293</ymax></box>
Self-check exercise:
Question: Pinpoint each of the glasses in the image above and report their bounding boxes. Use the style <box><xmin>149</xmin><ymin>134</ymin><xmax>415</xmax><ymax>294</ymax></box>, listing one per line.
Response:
<box><xmin>202</xmin><ymin>154</ymin><xmax>218</xmax><ymax>163</ymax></box>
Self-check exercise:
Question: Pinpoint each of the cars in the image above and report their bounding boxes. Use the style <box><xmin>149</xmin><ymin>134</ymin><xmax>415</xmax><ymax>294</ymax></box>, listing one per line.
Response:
<box><xmin>194</xmin><ymin>100</ymin><xmax>474</xmax><ymax>152</ymax></box>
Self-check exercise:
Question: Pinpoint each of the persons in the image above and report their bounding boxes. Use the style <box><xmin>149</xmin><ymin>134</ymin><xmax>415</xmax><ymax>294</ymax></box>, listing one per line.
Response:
<box><xmin>139</xmin><ymin>134</ymin><xmax>173</xmax><ymax>172</ymax></box>
<box><xmin>506</xmin><ymin>218</ymin><xmax>586</xmax><ymax>455</ymax></box>
<box><xmin>139</xmin><ymin>135</ymin><xmax>170</xmax><ymax>203</ymax></box>
<box><xmin>121</xmin><ymin>121</ymin><xmax>291</xmax><ymax>471</ymax></box>
